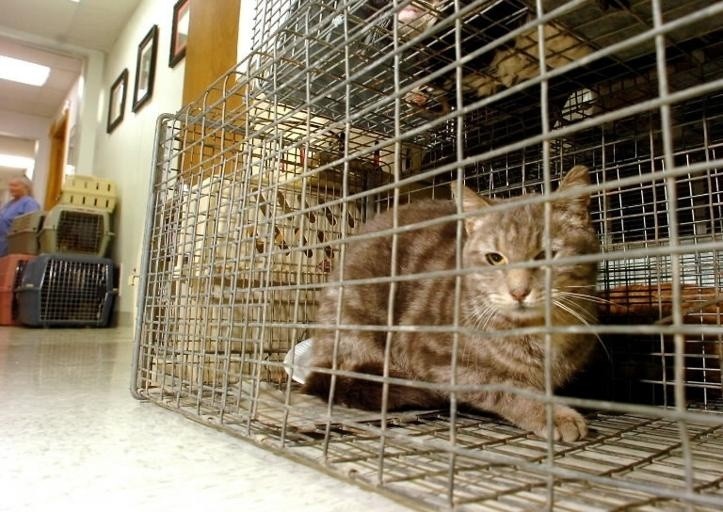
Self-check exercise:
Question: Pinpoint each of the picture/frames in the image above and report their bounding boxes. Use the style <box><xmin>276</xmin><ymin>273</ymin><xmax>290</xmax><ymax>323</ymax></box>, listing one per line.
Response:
<box><xmin>106</xmin><ymin>0</ymin><xmax>192</xmax><ymax>134</ymax></box>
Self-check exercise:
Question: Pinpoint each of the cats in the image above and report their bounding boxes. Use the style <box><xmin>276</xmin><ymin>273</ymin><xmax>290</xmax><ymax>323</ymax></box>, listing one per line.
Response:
<box><xmin>297</xmin><ymin>164</ymin><xmax>607</xmax><ymax>442</ymax></box>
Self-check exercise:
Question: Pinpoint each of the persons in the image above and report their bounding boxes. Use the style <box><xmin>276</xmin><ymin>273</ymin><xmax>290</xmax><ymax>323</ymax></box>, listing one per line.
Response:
<box><xmin>0</xmin><ymin>174</ymin><xmax>40</xmax><ymax>257</ymax></box>
